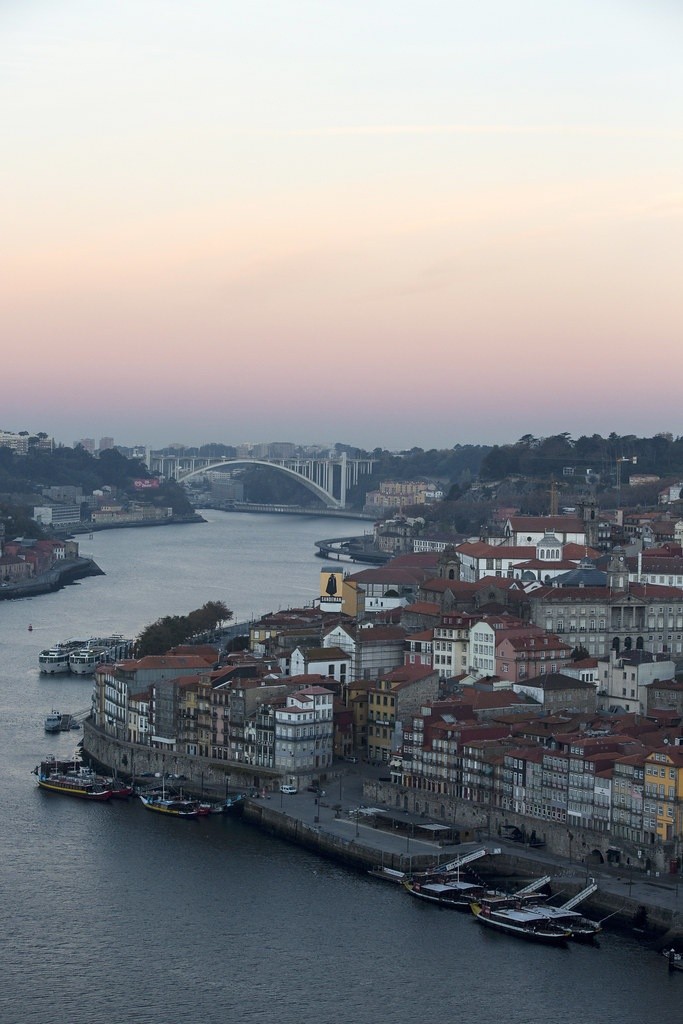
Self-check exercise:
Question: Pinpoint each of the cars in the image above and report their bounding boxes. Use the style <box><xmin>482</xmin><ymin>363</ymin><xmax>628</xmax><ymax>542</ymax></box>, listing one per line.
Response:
<box><xmin>344</xmin><ymin>756</ymin><xmax>359</xmax><ymax>764</ymax></box>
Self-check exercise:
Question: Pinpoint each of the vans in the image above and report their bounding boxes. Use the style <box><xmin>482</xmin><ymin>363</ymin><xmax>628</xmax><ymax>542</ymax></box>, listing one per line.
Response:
<box><xmin>280</xmin><ymin>785</ymin><xmax>297</xmax><ymax>795</ymax></box>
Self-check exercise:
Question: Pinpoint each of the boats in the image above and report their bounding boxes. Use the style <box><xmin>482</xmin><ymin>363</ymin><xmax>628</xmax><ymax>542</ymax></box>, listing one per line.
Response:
<box><xmin>45</xmin><ymin>708</ymin><xmax>78</xmax><ymax>731</ymax></box>
<box><xmin>69</xmin><ymin>634</ymin><xmax>136</xmax><ymax>676</ymax></box>
<box><xmin>404</xmin><ymin>851</ymin><xmax>604</xmax><ymax>944</ymax></box>
<box><xmin>38</xmin><ymin>639</ymin><xmax>90</xmax><ymax>674</ymax></box>
<box><xmin>36</xmin><ymin>755</ymin><xmax>228</xmax><ymax>816</ymax></box>
